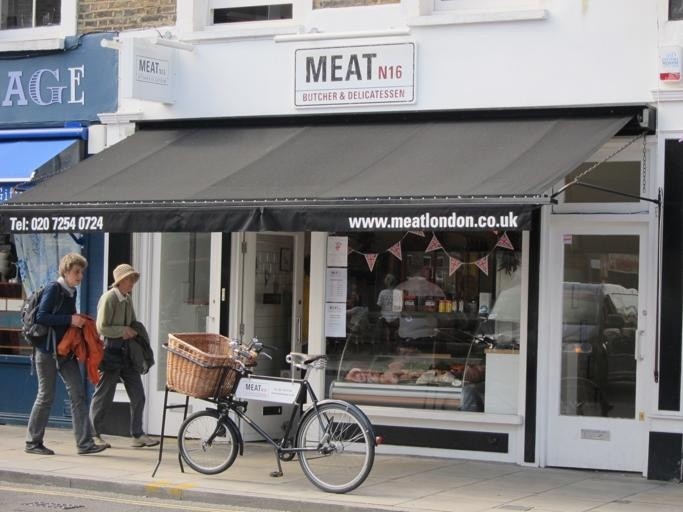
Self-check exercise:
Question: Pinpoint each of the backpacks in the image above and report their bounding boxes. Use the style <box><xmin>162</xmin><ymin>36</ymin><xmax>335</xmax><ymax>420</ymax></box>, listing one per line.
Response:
<box><xmin>19</xmin><ymin>280</ymin><xmax>65</xmax><ymax>376</ymax></box>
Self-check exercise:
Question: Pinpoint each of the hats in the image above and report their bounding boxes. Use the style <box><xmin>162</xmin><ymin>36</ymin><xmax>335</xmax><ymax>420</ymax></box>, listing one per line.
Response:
<box><xmin>112</xmin><ymin>264</ymin><xmax>139</xmax><ymax>286</ymax></box>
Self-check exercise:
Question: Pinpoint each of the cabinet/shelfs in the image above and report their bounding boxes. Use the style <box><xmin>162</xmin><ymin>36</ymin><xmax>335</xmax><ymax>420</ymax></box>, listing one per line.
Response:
<box><xmin>330</xmin><ymin>309</ymin><xmax>488</xmax><ymax>410</ymax></box>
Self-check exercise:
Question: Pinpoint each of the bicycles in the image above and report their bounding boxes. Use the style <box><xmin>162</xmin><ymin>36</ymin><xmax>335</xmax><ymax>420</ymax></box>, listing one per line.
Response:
<box><xmin>176</xmin><ymin>337</ymin><xmax>382</xmax><ymax>493</ymax></box>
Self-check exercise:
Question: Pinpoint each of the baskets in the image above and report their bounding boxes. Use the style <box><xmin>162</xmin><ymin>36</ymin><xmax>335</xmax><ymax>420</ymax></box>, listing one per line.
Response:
<box><xmin>166</xmin><ymin>332</ymin><xmax>259</xmax><ymax>398</ymax></box>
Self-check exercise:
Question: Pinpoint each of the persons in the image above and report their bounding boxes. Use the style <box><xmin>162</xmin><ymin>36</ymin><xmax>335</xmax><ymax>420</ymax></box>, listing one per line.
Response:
<box><xmin>22</xmin><ymin>252</ymin><xmax>108</xmax><ymax>453</ymax></box>
<box><xmin>377</xmin><ymin>274</ymin><xmax>400</xmax><ymax>335</ymax></box>
<box><xmin>378</xmin><ymin>261</ymin><xmax>451</xmax><ymax>352</ymax></box>
<box><xmin>84</xmin><ymin>263</ymin><xmax>158</xmax><ymax>448</ymax></box>
<box><xmin>344</xmin><ymin>299</ymin><xmax>370</xmax><ymax>332</ymax></box>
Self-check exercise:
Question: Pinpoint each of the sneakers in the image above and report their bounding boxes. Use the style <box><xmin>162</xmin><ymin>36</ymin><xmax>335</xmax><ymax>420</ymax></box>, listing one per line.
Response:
<box><xmin>25</xmin><ymin>441</ymin><xmax>54</xmax><ymax>455</ymax></box>
<box><xmin>131</xmin><ymin>434</ymin><xmax>160</xmax><ymax>447</ymax></box>
<box><xmin>78</xmin><ymin>436</ymin><xmax>111</xmax><ymax>455</ymax></box>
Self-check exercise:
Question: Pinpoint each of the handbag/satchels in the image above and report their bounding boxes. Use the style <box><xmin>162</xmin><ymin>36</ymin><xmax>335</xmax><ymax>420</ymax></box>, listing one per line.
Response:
<box><xmin>104</xmin><ymin>351</ymin><xmax>121</xmax><ymax>370</ymax></box>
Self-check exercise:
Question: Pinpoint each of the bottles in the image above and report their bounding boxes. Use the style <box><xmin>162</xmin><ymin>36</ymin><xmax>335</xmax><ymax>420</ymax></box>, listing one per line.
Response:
<box><xmin>438</xmin><ymin>297</ymin><xmax>464</xmax><ymax>314</ymax></box>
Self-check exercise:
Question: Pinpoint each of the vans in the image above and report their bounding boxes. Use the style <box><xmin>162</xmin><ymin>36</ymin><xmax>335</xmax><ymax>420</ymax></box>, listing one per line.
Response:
<box><xmin>459</xmin><ymin>279</ymin><xmax>638</xmax><ymax>417</ymax></box>
